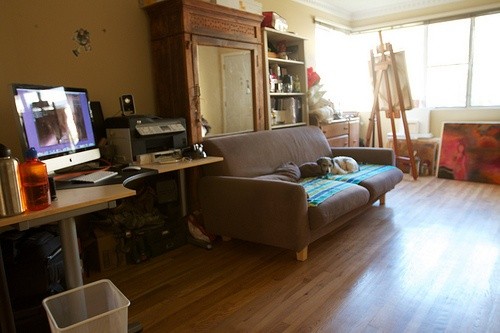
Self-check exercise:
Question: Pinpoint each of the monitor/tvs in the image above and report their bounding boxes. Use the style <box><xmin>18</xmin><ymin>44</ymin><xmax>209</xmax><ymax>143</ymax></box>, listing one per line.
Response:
<box><xmin>11</xmin><ymin>84</ymin><xmax>102</xmax><ymax>177</ymax></box>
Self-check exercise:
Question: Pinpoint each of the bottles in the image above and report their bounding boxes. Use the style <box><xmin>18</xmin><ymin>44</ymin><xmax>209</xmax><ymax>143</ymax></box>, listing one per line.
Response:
<box><xmin>19</xmin><ymin>147</ymin><xmax>51</xmax><ymax>211</ymax></box>
<box><xmin>283</xmin><ymin>74</ymin><xmax>300</xmax><ymax>93</ymax></box>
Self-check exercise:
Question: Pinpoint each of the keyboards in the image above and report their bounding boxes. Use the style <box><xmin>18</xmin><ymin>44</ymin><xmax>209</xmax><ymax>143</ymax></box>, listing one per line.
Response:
<box><xmin>70</xmin><ymin>170</ymin><xmax>118</xmax><ymax>184</ymax></box>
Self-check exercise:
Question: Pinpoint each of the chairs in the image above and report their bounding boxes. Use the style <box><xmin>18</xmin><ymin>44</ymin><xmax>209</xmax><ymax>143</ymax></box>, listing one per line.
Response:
<box><xmin>196</xmin><ymin>125</ymin><xmax>404</xmax><ymax>261</ymax></box>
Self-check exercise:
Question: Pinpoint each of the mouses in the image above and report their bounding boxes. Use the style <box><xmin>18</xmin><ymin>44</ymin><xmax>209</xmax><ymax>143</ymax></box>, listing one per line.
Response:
<box><xmin>122</xmin><ymin>166</ymin><xmax>141</xmax><ymax>172</ymax></box>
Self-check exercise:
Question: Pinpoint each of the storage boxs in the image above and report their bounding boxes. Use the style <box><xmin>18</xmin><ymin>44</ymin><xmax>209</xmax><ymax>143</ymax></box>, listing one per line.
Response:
<box><xmin>202</xmin><ymin>0</ymin><xmax>262</xmax><ymax>16</ymax></box>
<box><xmin>42</xmin><ymin>279</ymin><xmax>130</xmax><ymax>333</ymax></box>
<box><xmin>85</xmin><ymin>224</ymin><xmax>169</xmax><ymax>272</ymax></box>
<box><xmin>261</xmin><ymin>12</ymin><xmax>288</xmax><ymax>32</ymax></box>
<box><xmin>155</xmin><ymin>178</ymin><xmax>178</xmax><ymax>203</ymax></box>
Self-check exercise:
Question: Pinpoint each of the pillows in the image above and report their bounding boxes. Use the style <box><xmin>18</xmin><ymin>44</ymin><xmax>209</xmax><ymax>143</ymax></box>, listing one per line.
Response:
<box><xmin>256</xmin><ymin>162</ymin><xmax>301</xmax><ymax>183</ymax></box>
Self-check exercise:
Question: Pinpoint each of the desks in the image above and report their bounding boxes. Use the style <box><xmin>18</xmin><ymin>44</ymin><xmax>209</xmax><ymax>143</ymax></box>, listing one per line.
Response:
<box><xmin>130</xmin><ymin>156</ymin><xmax>224</xmax><ymax>250</ymax></box>
<box><xmin>0</xmin><ymin>184</ymin><xmax>136</xmax><ymax>333</ymax></box>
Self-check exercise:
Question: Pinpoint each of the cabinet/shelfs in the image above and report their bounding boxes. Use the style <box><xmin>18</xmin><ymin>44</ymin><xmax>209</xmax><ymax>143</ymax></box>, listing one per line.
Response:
<box><xmin>311</xmin><ymin>118</ymin><xmax>359</xmax><ymax>148</ymax></box>
<box><xmin>141</xmin><ymin>0</ymin><xmax>310</xmax><ymax>144</ymax></box>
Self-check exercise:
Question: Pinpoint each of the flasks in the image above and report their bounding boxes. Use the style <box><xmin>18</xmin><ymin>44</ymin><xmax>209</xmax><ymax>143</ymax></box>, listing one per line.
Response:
<box><xmin>0</xmin><ymin>144</ymin><xmax>28</xmax><ymax>217</ymax></box>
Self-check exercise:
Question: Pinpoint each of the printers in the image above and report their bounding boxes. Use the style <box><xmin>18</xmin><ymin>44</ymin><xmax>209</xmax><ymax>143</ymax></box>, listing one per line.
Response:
<box><xmin>103</xmin><ymin>116</ymin><xmax>189</xmax><ymax>167</ymax></box>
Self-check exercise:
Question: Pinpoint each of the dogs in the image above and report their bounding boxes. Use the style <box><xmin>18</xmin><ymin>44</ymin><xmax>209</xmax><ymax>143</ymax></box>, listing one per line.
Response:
<box><xmin>328</xmin><ymin>155</ymin><xmax>360</xmax><ymax>174</ymax></box>
<box><xmin>298</xmin><ymin>156</ymin><xmax>334</xmax><ymax>180</ymax></box>
